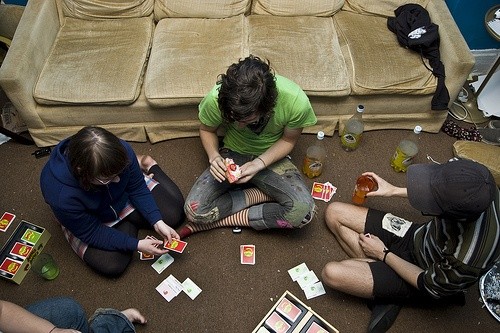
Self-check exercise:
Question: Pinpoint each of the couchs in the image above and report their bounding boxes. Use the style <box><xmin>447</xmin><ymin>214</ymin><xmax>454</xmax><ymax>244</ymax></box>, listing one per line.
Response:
<box><xmin>0</xmin><ymin>0</ymin><xmax>477</xmax><ymax>147</ymax></box>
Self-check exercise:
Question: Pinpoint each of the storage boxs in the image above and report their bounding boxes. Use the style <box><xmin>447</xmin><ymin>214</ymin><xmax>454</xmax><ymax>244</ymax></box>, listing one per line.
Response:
<box><xmin>251</xmin><ymin>290</ymin><xmax>340</xmax><ymax>333</ymax></box>
<box><xmin>0</xmin><ymin>220</ymin><xmax>51</xmax><ymax>285</ymax></box>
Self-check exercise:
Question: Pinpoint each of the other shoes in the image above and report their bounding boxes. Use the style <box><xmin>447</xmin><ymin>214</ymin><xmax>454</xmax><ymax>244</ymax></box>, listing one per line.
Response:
<box><xmin>367</xmin><ymin>304</ymin><xmax>401</xmax><ymax>333</ymax></box>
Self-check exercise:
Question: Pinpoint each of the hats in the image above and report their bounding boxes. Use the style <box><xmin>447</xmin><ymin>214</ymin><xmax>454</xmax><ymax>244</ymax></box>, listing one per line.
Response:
<box><xmin>407</xmin><ymin>159</ymin><xmax>497</xmax><ymax>213</ymax></box>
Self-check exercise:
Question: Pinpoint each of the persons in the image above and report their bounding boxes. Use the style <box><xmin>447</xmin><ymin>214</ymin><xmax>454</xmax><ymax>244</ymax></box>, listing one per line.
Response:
<box><xmin>177</xmin><ymin>54</ymin><xmax>318</xmax><ymax>238</ymax></box>
<box><xmin>40</xmin><ymin>126</ymin><xmax>186</xmax><ymax>279</ymax></box>
<box><xmin>322</xmin><ymin>157</ymin><xmax>500</xmax><ymax>312</ymax></box>
<box><xmin>0</xmin><ymin>295</ymin><xmax>147</xmax><ymax>333</ymax></box>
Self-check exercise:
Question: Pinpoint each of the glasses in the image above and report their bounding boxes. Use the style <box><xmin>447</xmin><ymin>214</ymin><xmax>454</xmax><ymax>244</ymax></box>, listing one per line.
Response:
<box><xmin>226</xmin><ymin>110</ymin><xmax>259</xmax><ymax>125</ymax></box>
<box><xmin>96</xmin><ymin>160</ymin><xmax>132</xmax><ymax>185</ymax></box>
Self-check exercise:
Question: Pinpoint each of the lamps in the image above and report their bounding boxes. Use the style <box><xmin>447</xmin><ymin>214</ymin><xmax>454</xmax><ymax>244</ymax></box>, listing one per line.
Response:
<box><xmin>451</xmin><ymin>4</ymin><xmax>500</xmax><ymax>125</ymax></box>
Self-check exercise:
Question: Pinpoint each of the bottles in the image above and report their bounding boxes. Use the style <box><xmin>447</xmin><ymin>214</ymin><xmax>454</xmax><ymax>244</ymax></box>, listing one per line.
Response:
<box><xmin>340</xmin><ymin>105</ymin><xmax>365</xmax><ymax>152</ymax></box>
<box><xmin>303</xmin><ymin>131</ymin><xmax>326</xmax><ymax>178</ymax></box>
<box><xmin>391</xmin><ymin>125</ymin><xmax>422</xmax><ymax>173</ymax></box>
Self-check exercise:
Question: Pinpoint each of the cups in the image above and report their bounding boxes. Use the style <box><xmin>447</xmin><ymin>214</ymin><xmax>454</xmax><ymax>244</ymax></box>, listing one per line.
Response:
<box><xmin>31</xmin><ymin>252</ymin><xmax>59</xmax><ymax>280</ymax></box>
<box><xmin>351</xmin><ymin>176</ymin><xmax>375</xmax><ymax>205</ymax></box>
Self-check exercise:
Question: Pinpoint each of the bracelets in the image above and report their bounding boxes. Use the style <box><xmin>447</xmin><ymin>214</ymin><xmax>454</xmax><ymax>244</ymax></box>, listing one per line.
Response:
<box><xmin>210</xmin><ymin>155</ymin><xmax>221</xmax><ymax>164</ymax></box>
<box><xmin>48</xmin><ymin>326</ymin><xmax>57</xmax><ymax>333</ymax></box>
<box><xmin>382</xmin><ymin>248</ymin><xmax>391</xmax><ymax>263</ymax></box>
<box><xmin>257</xmin><ymin>157</ymin><xmax>266</xmax><ymax>168</ymax></box>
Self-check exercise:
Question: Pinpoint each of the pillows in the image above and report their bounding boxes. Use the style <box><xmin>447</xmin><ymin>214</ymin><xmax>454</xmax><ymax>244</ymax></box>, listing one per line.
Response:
<box><xmin>453</xmin><ymin>140</ymin><xmax>500</xmax><ymax>186</ymax></box>
